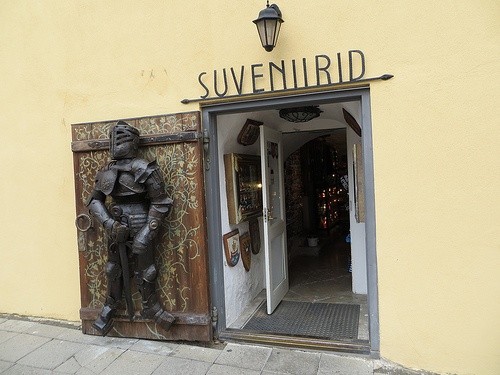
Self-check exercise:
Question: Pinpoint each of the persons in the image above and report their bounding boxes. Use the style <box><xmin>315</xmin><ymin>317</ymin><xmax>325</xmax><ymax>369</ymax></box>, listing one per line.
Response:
<box><xmin>86</xmin><ymin>121</ymin><xmax>174</xmax><ymax>337</ymax></box>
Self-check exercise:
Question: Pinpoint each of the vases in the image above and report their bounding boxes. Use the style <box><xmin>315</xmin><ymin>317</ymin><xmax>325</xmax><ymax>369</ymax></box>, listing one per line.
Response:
<box><xmin>308</xmin><ymin>237</ymin><xmax>318</xmax><ymax>246</ymax></box>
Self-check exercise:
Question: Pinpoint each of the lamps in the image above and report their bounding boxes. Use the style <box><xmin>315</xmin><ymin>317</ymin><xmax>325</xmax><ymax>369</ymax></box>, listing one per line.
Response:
<box><xmin>252</xmin><ymin>0</ymin><xmax>285</xmax><ymax>52</ymax></box>
<box><xmin>279</xmin><ymin>105</ymin><xmax>324</xmax><ymax>122</ymax></box>
